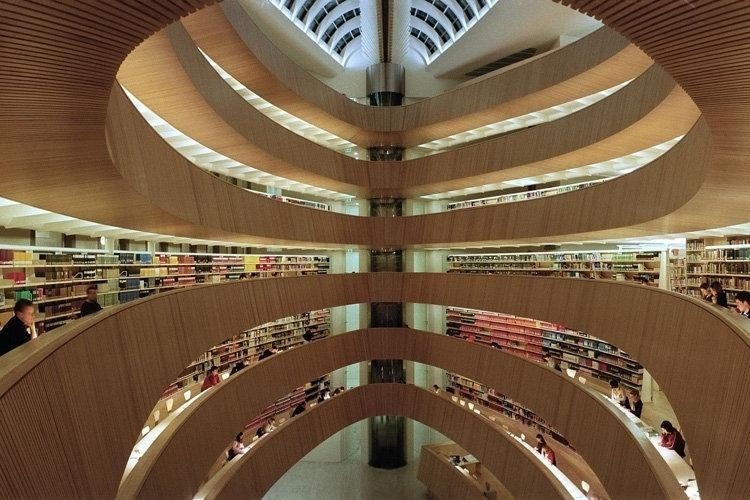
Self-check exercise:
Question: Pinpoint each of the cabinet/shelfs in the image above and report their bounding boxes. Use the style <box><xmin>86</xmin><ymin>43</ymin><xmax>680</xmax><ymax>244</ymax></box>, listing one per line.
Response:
<box><xmin>1</xmin><ymin>175</ymin><xmax>750</xmax><ymax>453</ymax></box>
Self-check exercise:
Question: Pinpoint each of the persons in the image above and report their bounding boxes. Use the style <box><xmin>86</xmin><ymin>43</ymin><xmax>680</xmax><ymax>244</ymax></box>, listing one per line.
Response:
<box><xmin>232</xmin><ymin>431</ymin><xmax>245</xmax><ymax>455</ymax></box>
<box><xmin>609</xmin><ymin>379</ymin><xmax>643</xmax><ymax>417</ymax></box>
<box><xmin>660</xmin><ymin>420</ymin><xmax>686</xmax><ymax>457</ymax></box>
<box><xmin>481</xmin><ymin>481</ymin><xmax>497</xmax><ymax>500</ymax></box>
<box><xmin>734</xmin><ymin>291</ymin><xmax>750</xmax><ymax>319</ymax></box>
<box><xmin>332</xmin><ymin>386</ymin><xmax>344</xmax><ymax>396</ymax></box>
<box><xmin>0</xmin><ymin>298</ymin><xmax>37</xmax><ymax>356</ymax></box>
<box><xmin>433</xmin><ymin>384</ymin><xmax>441</xmax><ymax>396</ymax></box>
<box><xmin>536</xmin><ymin>433</ymin><xmax>555</xmax><ymax>465</ymax></box>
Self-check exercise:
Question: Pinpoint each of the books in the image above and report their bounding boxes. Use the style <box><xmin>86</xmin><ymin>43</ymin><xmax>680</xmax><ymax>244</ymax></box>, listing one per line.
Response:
<box><xmin>158</xmin><ymin>308</ymin><xmax>332</xmax><ymax>433</ymax></box>
<box><xmin>0</xmin><ymin>249</ymin><xmax>330</xmax><ymax>336</ymax></box>
<box><xmin>446</xmin><ymin>236</ymin><xmax>750</xmax><ymax>306</ymax></box>
<box><xmin>445</xmin><ymin>306</ymin><xmax>643</xmax><ymax>391</ymax></box>
<box><xmin>445</xmin><ymin>371</ymin><xmax>576</xmax><ymax>454</ymax></box>
